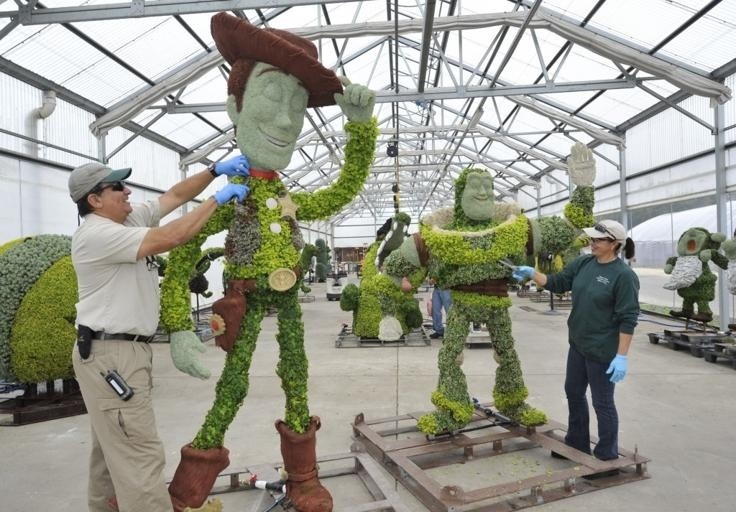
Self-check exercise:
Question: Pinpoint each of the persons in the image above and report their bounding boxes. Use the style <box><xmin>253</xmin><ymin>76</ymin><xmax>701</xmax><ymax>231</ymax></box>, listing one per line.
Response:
<box><xmin>665</xmin><ymin>226</ymin><xmax>727</xmax><ymax>321</ymax></box>
<box><xmin>64</xmin><ymin>153</ymin><xmax>250</xmax><ymax>512</ymax></box>
<box><xmin>384</xmin><ymin>141</ymin><xmax>600</xmax><ymax>434</ymax></box>
<box><xmin>429</xmin><ymin>274</ymin><xmax>453</xmax><ymax>339</ymax></box>
<box><xmin>107</xmin><ymin>13</ymin><xmax>375</xmax><ymax>512</ymax></box>
<box><xmin>512</xmin><ymin>221</ymin><xmax>640</xmax><ymax>479</ymax></box>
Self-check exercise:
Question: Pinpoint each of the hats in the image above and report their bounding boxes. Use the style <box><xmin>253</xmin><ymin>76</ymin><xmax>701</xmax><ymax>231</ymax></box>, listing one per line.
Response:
<box><xmin>68</xmin><ymin>162</ymin><xmax>131</xmax><ymax>203</ymax></box>
<box><xmin>584</xmin><ymin>220</ymin><xmax>626</xmax><ymax>245</ymax></box>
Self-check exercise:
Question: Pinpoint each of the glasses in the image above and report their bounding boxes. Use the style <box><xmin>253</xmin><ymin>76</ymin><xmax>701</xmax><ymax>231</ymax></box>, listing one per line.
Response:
<box><xmin>102</xmin><ymin>184</ymin><xmax>124</xmax><ymax>192</ymax></box>
<box><xmin>595</xmin><ymin>224</ymin><xmax>616</xmax><ymax>239</ymax></box>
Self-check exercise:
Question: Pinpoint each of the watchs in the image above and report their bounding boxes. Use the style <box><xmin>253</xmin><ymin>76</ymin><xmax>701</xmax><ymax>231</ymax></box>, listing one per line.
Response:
<box><xmin>208</xmin><ymin>162</ymin><xmax>219</xmax><ymax>177</ymax></box>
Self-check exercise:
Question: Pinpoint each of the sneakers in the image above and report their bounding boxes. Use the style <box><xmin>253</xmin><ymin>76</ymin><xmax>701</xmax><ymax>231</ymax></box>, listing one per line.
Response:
<box><xmin>582</xmin><ymin>468</ymin><xmax>619</xmax><ymax>480</ymax></box>
<box><xmin>429</xmin><ymin>333</ymin><xmax>442</xmax><ymax>338</ymax></box>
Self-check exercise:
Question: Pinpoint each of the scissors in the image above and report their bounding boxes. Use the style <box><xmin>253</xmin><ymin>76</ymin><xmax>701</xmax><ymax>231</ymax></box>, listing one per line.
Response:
<box><xmin>499</xmin><ymin>257</ymin><xmax>517</xmax><ymax>270</ymax></box>
<box><xmin>231</xmin><ymin>175</ymin><xmax>251</xmax><ymax>205</ymax></box>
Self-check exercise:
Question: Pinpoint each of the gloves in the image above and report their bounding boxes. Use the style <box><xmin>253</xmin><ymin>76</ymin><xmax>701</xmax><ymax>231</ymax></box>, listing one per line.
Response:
<box><xmin>215</xmin><ymin>156</ymin><xmax>250</xmax><ymax>178</ymax></box>
<box><xmin>215</xmin><ymin>185</ymin><xmax>248</xmax><ymax>205</ymax></box>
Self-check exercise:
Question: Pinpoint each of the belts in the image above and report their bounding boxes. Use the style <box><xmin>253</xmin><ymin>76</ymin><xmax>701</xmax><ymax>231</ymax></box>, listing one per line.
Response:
<box><xmin>605</xmin><ymin>355</ymin><xmax>627</xmax><ymax>383</ymax></box>
<box><xmin>512</xmin><ymin>266</ymin><xmax>535</xmax><ymax>284</ymax></box>
<box><xmin>93</xmin><ymin>330</ymin><xmax>154</xmax><ymax>342</ymax></box>
